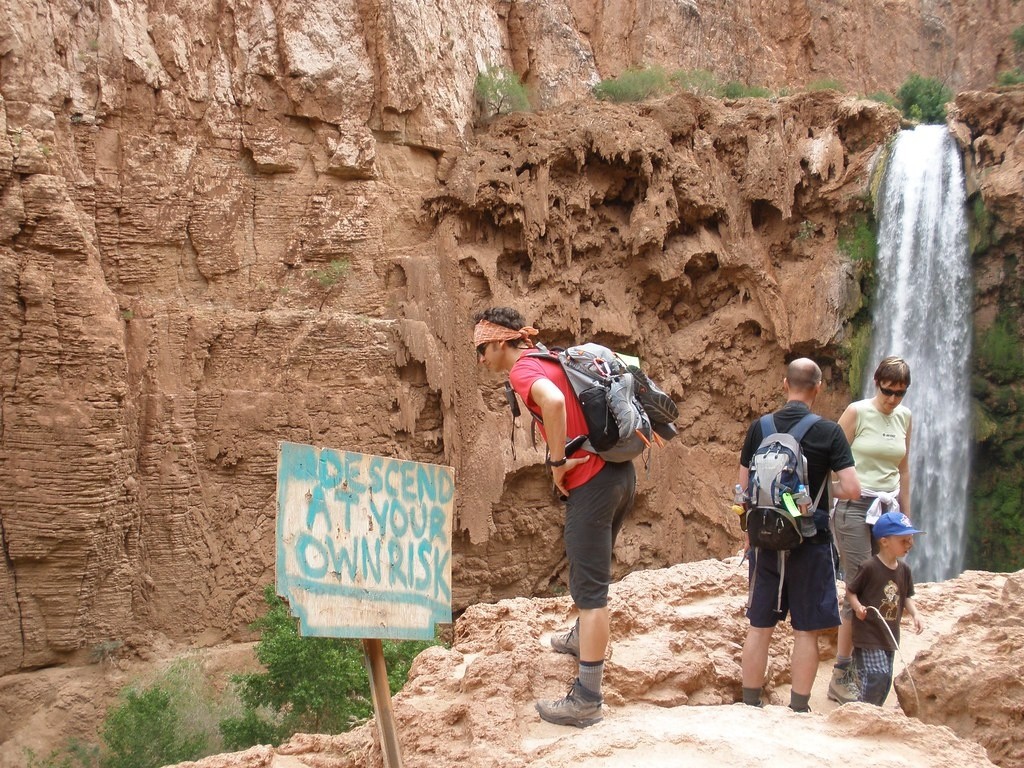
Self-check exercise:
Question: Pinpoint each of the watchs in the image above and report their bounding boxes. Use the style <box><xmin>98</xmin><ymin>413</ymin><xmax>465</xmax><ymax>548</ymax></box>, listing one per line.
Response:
<box><xmin>545</xmin><ymin>450</ymin><xmax>568</xmax><ymax>467</ymax></box>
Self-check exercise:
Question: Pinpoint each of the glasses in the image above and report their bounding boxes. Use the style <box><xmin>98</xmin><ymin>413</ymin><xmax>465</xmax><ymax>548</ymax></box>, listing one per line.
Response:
<box><xmin>878</xmin><ymin>381</ymin><xmax>907</xmax><ymax>397</ymax></box>
<box><xmin>475</xmin><ymin>342</ymin><xmax>491</xmax><ymax>356</ymax></box>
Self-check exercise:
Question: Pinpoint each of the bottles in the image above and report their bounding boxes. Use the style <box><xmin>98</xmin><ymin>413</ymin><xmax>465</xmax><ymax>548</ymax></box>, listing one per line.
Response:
<box><xmin>796</xmin><ymin>484</ymin><xmax>817</xmax><ymax>537</ymax></box>
<box><xmin>733</xmin><ymin>485</ymin><xmax>749</xmax><ymax>514</ymax></box>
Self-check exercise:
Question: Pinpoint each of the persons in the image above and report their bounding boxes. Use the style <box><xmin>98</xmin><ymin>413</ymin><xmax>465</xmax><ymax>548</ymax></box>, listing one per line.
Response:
<box><xmin>475</xmin><ymin>306</ymin><xmax>638</xmax><ymax>729</ymax></box>
<box><xmin>847</xmin><ymin>513</ymin><xmax>923</xmax><ymax>706</ymax></box>
<box><xmin>736</xmin><ymin>358</ymin><xmax>859</xmax><ymax>713</ymax></box>
<box><xmin>830</xmin><ymin>355</ymin><xmax>914</xmax><ymax>703</ymax></box>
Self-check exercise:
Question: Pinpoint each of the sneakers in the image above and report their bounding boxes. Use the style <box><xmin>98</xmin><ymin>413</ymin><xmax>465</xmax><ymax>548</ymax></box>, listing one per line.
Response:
<box><xmin>536</xmin><ymin>694</ymin><xmax>604</xmax><ymax>729</ymax></box>
<box><xmin>551</xmin><ymin>628</ymin><xmax>580</xmax><ymax>657</ymax></box>
<box><xmin>827</xmin><ymin>666</ymin><xmax>863</xmax><ymax>705</ymax></box>
<box><xmin>602</xmin><ymin>372</ymin><xmax>636</xmax><ymax>438</ymax></box>
<box><xmin>622</xmin><ymin>364</ymin><xmax>679</xmax><ymax>423</ymax></box>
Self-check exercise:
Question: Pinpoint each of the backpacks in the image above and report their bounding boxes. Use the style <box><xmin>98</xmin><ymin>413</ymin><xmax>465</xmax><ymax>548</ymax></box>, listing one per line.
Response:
<box><xmin>746</xmin><ymin>414</ymin><xmax>835</xmax><ymax>552</ymax></box>
<box><xmin>516</xmin><ymin>340</ymin><xmax>652</xmax><ymax>464</ymax></box>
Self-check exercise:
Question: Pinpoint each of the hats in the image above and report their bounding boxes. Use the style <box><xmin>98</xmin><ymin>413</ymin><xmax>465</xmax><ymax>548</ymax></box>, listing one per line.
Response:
<box><xmin>872</xmin><ymin>511</ymin><xmax>928</xmax><ymax>541</ymax></box>
